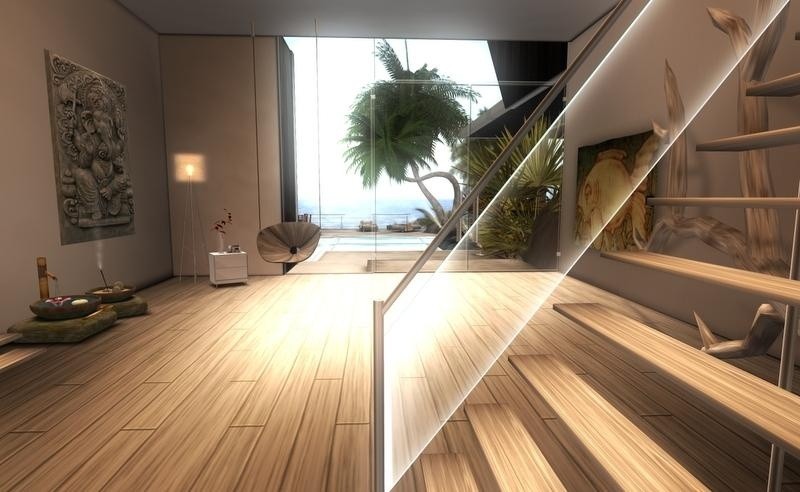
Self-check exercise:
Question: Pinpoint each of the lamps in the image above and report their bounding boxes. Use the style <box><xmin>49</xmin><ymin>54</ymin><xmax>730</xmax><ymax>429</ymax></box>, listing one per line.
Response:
<box><xmin>173</xmin><ymin>154</ymin><xmax>207</xmax><ymax>284</ymax></box>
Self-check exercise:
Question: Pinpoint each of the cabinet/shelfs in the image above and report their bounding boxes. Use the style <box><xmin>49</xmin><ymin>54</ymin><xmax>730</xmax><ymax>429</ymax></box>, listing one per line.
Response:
<box><xmin>208</xmin><ymin>250</ymin><xmax>248</xmax><ymax>289</ymax></box>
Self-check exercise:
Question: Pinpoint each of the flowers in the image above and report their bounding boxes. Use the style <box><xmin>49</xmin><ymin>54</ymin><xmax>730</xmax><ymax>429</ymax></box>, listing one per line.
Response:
<box><xmin>209</xmin><ymin>208</ymin><xmax>233</xmax><ymax>234</ymax></box>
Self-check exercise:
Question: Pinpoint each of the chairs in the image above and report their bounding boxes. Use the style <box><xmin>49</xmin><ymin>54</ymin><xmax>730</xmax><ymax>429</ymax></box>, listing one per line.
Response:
<box><xmin>359</xmin><ymin>220</ymin><xmax>422</xmax><ymax>231</ymax></box>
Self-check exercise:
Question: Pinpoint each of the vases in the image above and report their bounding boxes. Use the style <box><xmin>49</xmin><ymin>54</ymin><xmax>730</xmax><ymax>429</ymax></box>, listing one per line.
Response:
<box><xmin>217</xmin><ymin>234</ymin><xmax>223</xmax><ymax>252</ymax></box>
